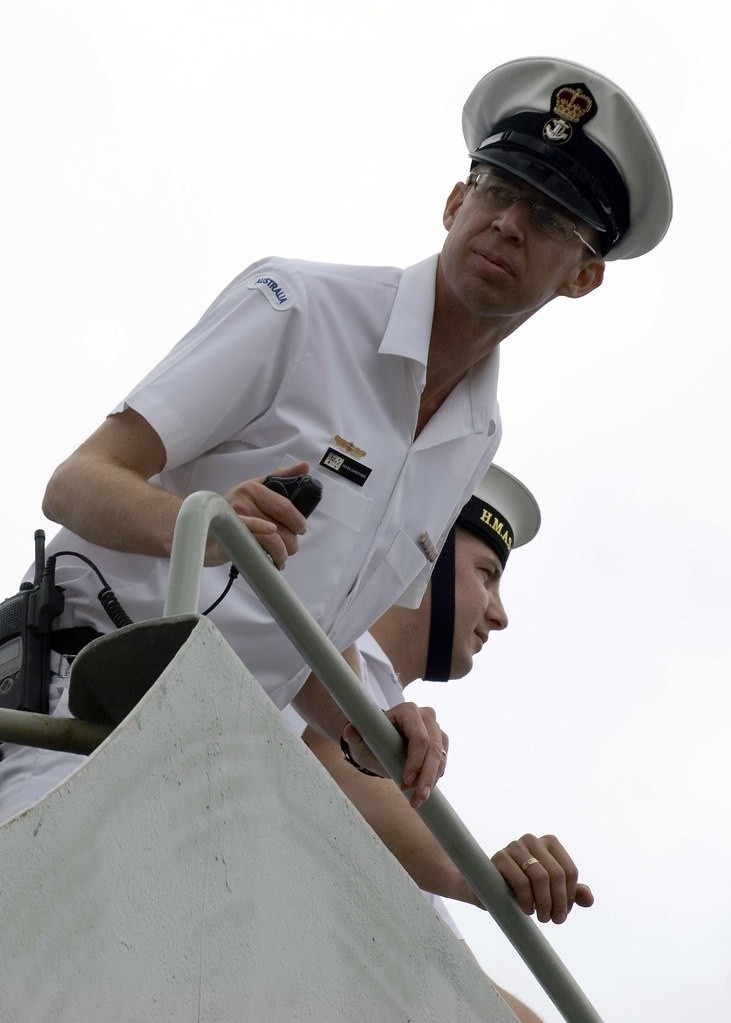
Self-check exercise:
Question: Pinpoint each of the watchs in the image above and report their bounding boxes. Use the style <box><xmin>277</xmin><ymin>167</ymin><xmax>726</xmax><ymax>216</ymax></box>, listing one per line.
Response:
<box><xmin>336</xmin><ymin>713</ymin><xmax>385</xmax><ymax>782</ymax></box>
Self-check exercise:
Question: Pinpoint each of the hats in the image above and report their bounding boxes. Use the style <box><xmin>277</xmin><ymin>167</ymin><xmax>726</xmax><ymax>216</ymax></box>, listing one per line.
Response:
<box><xmin>461</xmin><ymin>55</ymin><xmax>673</xmax><ymax>262</ymax></box>
<box><xmin>456</xmin><ymin>461</ymin><xmax>543</xmax><ymax>572</ymax></box>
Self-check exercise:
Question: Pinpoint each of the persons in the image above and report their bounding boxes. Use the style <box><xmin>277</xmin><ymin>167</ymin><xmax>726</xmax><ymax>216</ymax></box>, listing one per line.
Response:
<box><xmin>1</xmin><ymin>56</ymin><xmax>672</xmax><ymax>1023</ymax></box>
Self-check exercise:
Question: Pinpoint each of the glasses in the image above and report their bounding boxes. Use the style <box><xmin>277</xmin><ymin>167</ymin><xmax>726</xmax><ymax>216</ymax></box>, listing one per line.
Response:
<box><xmin>469</xmin><ymin>170</ymin><xmax>598</xmax><ymax>257</ymax></box>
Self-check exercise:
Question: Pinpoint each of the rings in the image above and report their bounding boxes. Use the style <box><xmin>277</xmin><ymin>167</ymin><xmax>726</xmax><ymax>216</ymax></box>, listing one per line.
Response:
<box><xmin>521</xmin><ymin>857</ymin><xmax>539</xmax><ymax>872</ymax></box>
<box><xmin>442</xmin><ymin>748</ymin><xmax>448</xmax><ymax>757</ymax></box>
